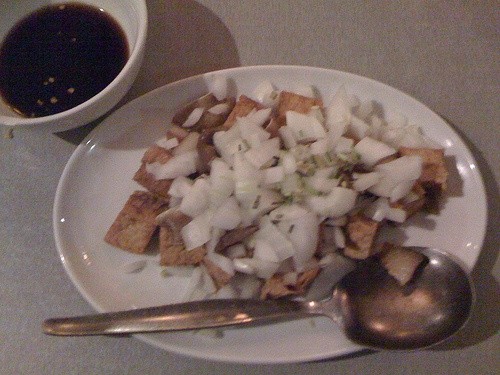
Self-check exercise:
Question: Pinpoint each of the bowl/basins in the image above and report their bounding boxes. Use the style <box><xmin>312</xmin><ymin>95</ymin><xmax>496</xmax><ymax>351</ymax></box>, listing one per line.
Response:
<box><xmin>1</xmin><ymin>0</ymin><xmax>148</xmax><ymax>137</ymax></box>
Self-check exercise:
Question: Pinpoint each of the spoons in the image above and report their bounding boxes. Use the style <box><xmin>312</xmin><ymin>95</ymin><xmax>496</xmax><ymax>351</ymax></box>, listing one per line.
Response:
<box><xmin>43</xmin><ymin>246</ymin><xmax>476</xmax><ymax>349</ymax></box>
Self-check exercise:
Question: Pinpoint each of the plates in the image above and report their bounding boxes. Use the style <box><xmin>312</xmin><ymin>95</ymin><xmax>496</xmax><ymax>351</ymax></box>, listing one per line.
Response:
<box><xmin>49</xmin><ymin>61</ymin><xmax>487</xmax><ymax>366</ymax></box>
